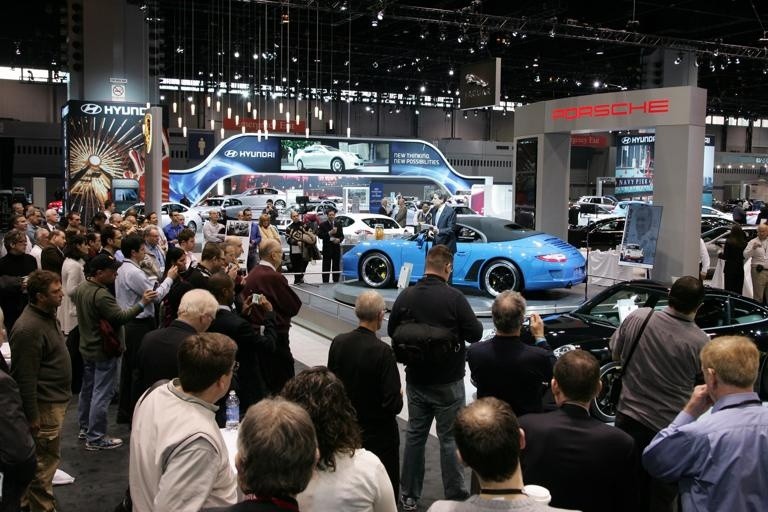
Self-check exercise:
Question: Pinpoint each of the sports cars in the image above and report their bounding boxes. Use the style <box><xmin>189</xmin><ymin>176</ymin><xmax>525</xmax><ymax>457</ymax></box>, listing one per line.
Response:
<box><xmin>337</xmin><ymin>216</ymin><xmax>588</xmax><ymax>302</ymax></box>
<box><xmin>120</xmin><ymin>185</ymin><xmax>417</xmax><ymax>287</ymax></box>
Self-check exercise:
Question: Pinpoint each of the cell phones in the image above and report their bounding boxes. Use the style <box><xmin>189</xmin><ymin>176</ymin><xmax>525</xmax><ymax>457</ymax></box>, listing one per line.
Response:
<box><xmin>152</xmin><ymin>282</ymin><xmax>158</xmax><ymax>291</ymax></box>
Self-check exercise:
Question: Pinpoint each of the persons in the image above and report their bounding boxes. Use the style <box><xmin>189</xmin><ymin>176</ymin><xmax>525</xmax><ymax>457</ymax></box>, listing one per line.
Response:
<box><xmin>413</xmin><ymin>202</ymin><xmax>432</xmax><ymax>226</ymax></box>
<box><xmin>233</xmin><ymin>277</ymin><xmax>767</xmax><ymax>511</ymax></box>
<box><xmin>317</xmin><ymin>208</ymin><xmax>344</xmax><ymax>283</ymax></box>
<box><xmin>0</xmin><ymin>195</ymin><xmax>308</xmax><ymax>512</ymax></box>
<box><xmin>388</xmin><ymin>245</ymin><xmax>485</xmax><ymax>510</ymax></box>
<box><xmin>568</xmin><ymin>202</ymin><xmax>578</xmax><ymax>228</ymax></box>
<box><xmin>699</xmin><ymin>199</ymin><xmax>767</xmax><ymax>303</ymax></box>
<box><xmin>426</xmin><ymin>191</ymin><xmax>456</xmax><ymax>284</ymax></box>
<box><xmin>380</xmin><ymin>197</ymin><xmax>407</xmax><ymax>229</ymax></box>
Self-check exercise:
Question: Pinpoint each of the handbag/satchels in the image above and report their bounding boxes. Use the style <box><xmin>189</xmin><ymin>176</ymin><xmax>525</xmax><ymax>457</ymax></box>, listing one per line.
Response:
<box><xmin>392</xmin><ymin>321</ymin><xmax>463</xmax><ymax>369</ymax></box>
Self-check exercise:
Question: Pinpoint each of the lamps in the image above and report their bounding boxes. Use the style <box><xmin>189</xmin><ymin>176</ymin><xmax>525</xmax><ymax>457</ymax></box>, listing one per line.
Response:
<box><xmin>165</xmin><ymin>0</ymin><xmax>359</xmax><ymax>142</ymax></box>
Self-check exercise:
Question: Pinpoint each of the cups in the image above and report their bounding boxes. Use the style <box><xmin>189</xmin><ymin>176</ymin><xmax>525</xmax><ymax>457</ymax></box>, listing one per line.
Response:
<box><xmin>521</xmin><ymin>484</ymin><xmax>551</xmax><ymax>505</ymax></box>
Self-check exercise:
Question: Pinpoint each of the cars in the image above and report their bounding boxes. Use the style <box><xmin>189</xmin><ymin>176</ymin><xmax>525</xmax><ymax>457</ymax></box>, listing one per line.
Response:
<box><xmin>46</xmin><ymin>196</ymin><xmax>65</xmax><ymax>215</ymax></box>
<box><xmin>392</xmin><ymin>193</ymin><xmax>482</xmax><ymax>231</ymax></box>
<box><xmin>519</xmin><ymin>278</ymin><xmax>768</xmax><ymax>420</ymax></box>
<box><xmin>571</xmin><ymin>195</ymin><xmax>767</xmax><ymax>273</ymax></box>
<box><xmin>105</xmin><ymin>178</ymin><xmax>139</xmax><ymax>214</ymax></box>
<box><xmin>294</xmin><ymin>144</ymin><xmax>364</xmax><ymax>172</ymax></box>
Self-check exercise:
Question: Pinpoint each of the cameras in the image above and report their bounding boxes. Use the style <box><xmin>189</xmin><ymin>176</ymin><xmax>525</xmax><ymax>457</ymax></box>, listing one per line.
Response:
<box><xmin>252</xmin><ymin>294</ymin><xmax>259</xmax><ymax>302</ymax></box>
<box><xmin>521</xmin><ymin>317</ymin><xmax>529</xmax><ymax>326</ymax></box>
<box><xmin>228</xmin><ymin>263</ymin><xmax>233</xmax><ymax>270</ymax></box>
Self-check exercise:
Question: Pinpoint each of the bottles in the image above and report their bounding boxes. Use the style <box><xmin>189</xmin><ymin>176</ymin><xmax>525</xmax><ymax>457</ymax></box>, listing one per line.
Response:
<box><xmin>226</xmin><ymin>390</ymin><xmax>239</xmax><ymax>432</ymax></box>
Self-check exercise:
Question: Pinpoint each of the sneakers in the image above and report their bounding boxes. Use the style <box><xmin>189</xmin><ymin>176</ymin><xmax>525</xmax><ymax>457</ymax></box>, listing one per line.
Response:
<box><xmin>401</xmin><ymin>496</ymin><xmax>418</xmax><ymax>511</ymax></box>
<box><xmin>85</xmin><ymin>437</ymin><xmax>123</xmax><ymax>451</ymax></box>
